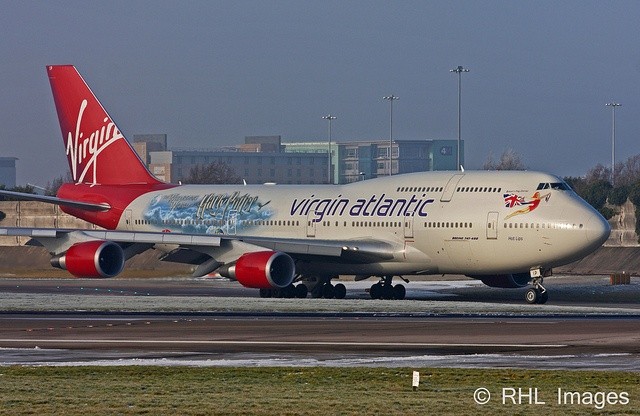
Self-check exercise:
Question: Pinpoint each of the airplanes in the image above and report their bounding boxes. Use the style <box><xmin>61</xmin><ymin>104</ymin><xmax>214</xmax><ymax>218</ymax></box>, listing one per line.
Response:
<box><xmin>1</xmin><ymin>65</ymin><xmax>609</xmax><ymax>304</ymax></box>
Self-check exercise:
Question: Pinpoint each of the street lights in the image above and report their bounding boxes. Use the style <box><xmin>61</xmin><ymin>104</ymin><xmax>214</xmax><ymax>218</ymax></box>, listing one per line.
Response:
<box><xmin>605</xmin><ymin>102</ymin><xmax>623</xmax><ymax>186</ymax></box>
<box><xmin>322</xmin><ymin>115</ymin><xmax>337</xmax><ymax>184</ymax></box>
<box><xmin>449</xmin><ymin>66</ymin><xmax>469</xmax><ymax>171</ymax></box>
<box><xmin>382</xmin><ymin>92</ymin><xmax>400</xmax><ymax>177</ymax></box>
